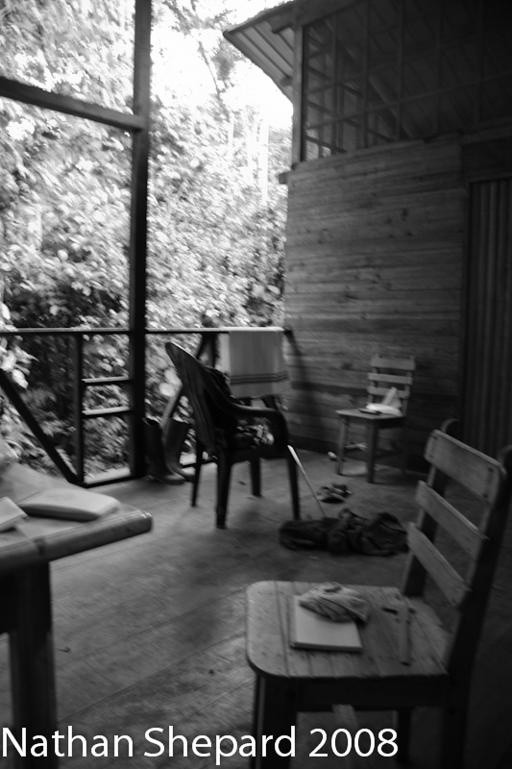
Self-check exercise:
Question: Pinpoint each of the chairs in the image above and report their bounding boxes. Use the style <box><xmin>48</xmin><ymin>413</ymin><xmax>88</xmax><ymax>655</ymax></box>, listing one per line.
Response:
<box><xmin>238</xmin><ymin>415</ymin><xmax>510</xmax><ymax>768</ymax></box>
<box><xmin>330</xmin><ymin>349</ymin><xmax>418</xmax><ymax>482</ymax></box>
<box><xmin>159</xmin><ymin>336</ymin><xmax>304</xmax><ymax>531</ymax></box>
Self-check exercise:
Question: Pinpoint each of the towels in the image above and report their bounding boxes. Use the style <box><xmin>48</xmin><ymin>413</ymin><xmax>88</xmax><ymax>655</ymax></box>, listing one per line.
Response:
<box><xmin>220</xmin><ymin>325</ymin><xmax>293</xmax><ymax>403</ymax></box>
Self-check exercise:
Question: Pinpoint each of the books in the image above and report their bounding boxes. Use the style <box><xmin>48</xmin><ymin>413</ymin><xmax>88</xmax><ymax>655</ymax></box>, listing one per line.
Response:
<box><xmin>287</xmin><ymin>594</ymin><xmax>363</xmax><ymax>653</ymax></box>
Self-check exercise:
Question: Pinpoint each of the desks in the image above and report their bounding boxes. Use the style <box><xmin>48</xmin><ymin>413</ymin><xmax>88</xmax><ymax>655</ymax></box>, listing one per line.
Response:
<box><xmin>1</xmin><ymin>465</ymin><xmax>154</xmax><ymax>769</ymax></box>
